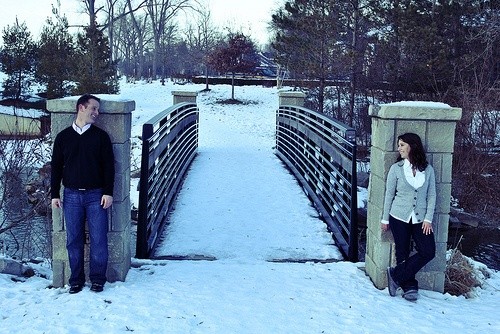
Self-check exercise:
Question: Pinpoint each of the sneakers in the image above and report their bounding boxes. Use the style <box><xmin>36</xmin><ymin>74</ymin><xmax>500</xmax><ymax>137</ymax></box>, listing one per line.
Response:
<box><xmin>387</xmin><ymin>267</ymin><xmax>400</xmax><ymax>296</ymax></box>
<box><xmin>402</xmin><ymin>286</ymin><xmax>418</xmax><ymax>300</ymax></box>
<box><xmin>90</xmin><ymin>283</ymin><xmax>103</xmax><ymax>292</ymax></box>
<box><xmin>69</xmin><ymin>281</ymin><xmax>86</xmax><ymax>293</ymax></box>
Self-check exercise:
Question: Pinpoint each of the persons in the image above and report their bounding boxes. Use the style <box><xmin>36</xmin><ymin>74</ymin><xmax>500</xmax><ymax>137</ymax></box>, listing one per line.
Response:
<box><xmin>50</xmin><ymin>94</ymin><xmax>115</xmax><ymax>293</ymax></box>
<box><xmin>381</xmin><ymin>132</ymin><xmax>436</xmax><ymax>300</ymax></box>
